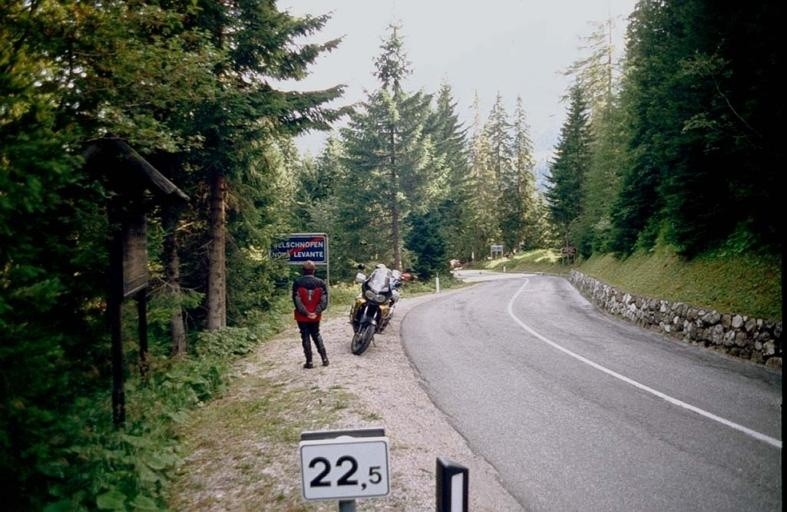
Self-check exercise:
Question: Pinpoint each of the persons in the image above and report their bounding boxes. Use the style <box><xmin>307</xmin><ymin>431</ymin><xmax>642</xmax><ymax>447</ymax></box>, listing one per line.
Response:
<box><xmin>291</xmin><ymin>259</ymin><xmax>329</xmax><ymax>368</ymax></box>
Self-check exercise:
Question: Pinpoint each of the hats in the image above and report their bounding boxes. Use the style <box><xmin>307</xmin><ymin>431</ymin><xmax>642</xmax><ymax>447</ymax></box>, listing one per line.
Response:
<box><xmin>304</xmin><ymin>260</ymin><xmax>319</xmax><ymax>270</ymax></box>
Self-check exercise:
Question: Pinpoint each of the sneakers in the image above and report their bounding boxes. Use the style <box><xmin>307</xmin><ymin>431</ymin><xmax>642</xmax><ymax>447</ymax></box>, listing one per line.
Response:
<box><xmin>323</xmin><ymin>358</ymin><xmax>328</xmax><ymax>366</ymax></box>
<box><xmin>304</xmin><ymin>362</ymin><xmax>312</xmax><ymax>368</ymax></box>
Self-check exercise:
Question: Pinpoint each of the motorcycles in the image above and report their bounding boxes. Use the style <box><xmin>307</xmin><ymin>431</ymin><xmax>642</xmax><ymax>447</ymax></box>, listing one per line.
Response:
<box><xmin>347</xmin><ymin>263</ymin><xmax>418</xmax><ymax>354</ymax></box>
